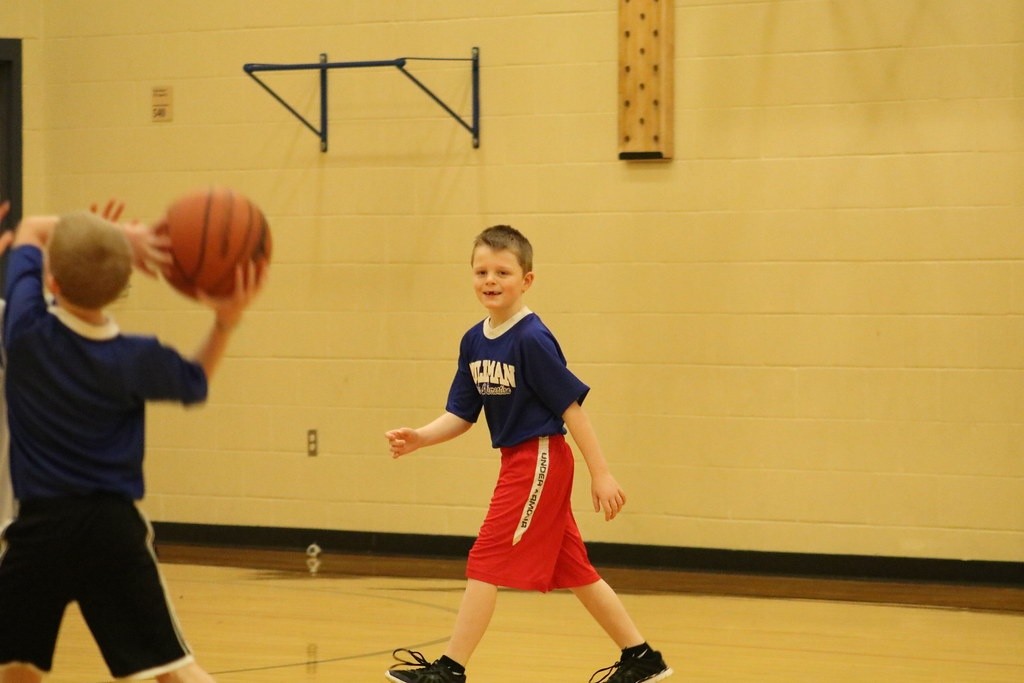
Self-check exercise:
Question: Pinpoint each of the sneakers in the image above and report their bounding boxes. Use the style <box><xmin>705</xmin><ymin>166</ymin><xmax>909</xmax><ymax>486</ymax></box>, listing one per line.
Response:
<box><xmin>602</xmin><ymin>651</ymin><xmax>675</xmax><ymax>683</ymax></box>
<box><xmin>385</xmin><ymin>648</ymin><xmax>467</xmax><ymax>683</ymax></box>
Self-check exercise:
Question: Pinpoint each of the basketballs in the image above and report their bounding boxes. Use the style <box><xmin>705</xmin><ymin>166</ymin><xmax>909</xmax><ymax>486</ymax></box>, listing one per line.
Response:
<box><xmin>155</xmin><ymin>189</ymin><xmax>272</xmax><ymax>301</ymax></box>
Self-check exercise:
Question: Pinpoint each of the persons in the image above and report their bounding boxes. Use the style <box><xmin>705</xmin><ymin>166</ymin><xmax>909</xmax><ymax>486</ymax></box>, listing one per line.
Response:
<box><xmin>0</xmin><ymin>193</ymin><xmax>272</xmax><ymax>683</ymax></box>
<box><xmin>386</xmin><ymin>222</ymin><xmax>675</xmax><ymax>683</ymax></box>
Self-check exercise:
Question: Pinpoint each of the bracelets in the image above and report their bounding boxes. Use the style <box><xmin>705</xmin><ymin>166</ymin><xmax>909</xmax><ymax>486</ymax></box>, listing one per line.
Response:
<box><xmin>214</xmin><ymin>317</ymin><xmax>239</xmax><ymax>333</ymax></box>
